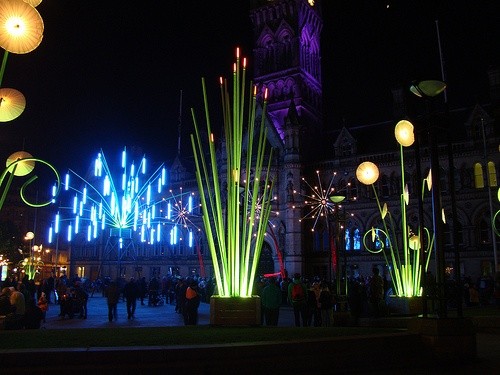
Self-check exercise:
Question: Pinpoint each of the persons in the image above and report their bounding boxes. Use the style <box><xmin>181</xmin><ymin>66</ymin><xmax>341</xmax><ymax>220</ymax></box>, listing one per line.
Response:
<box><xmin>432</xmin><ymin>272</ymin><xmax>467</xmax><ymax>311</ymax></box>
<box><xmin>125</xmin><ymin>278</ymin><xmax>136</xmax><ymax>319</ymax></box>
<box><xmin>342</xmin><ymin>275</ymin><xmax>389</xmax><ymax>317</ymax></box>
<box><xmin>182</xmin><ymin>282</ymin><xmax>201</xmax><ymax>326</ymax></box>
<box><xmin>104</xmin><ymin>275</ymin><xmax>174</xmax><ymax>305</ymax></box>
<box><xmin>255</xmin><ymin>270</ymin><xmax>336</xmax><ymax>327</ymax></box>
<box><xmin>470</xmin><ymin>287</ymin><xmax>478</xmax><ymax>311</ymax></box>
<box><xmin>0</xmin><ymin>271</ymin><xmax>89</xmax><ymax>330</ymax></box>
<box><xmin>175</xmin><ymin>276</ymin><xmax>216</xmax><ymax>313</ymax></box>
<box><xmin>106</xmin><ymin>281</ymin><xmax>118</xmax><ymax>321</ymax></box>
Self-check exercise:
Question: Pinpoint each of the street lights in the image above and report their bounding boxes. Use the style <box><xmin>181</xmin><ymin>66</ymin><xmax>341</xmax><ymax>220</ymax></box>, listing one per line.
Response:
<box><xmin>400</xmin><ymin>73</ymin><xmax>448</xmax><ymax>318</ymax></box>
<box><xmin>25</xmin><ymin>232</ymin><xmax>35</xmax><ymax>280</ymax></box>
<box><xmin>330</xmin><ymin>191</ymin><xmax>349</xmax><ymax>312</ymax></box>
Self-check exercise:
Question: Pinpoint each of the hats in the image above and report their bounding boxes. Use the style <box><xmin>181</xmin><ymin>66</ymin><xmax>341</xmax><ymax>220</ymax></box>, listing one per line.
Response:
<box><xmin>312</xmin><ymin>282</ymin><xmax>319</xmax><ymax>287</ymax></box>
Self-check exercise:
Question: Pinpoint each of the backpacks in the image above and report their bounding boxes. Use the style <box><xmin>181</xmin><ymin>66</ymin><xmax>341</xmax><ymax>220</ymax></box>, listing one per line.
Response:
<box><xmin>291</xmin><ymin>284</ymin><xmax>304</xmax><ymax>303</ymax></box>
